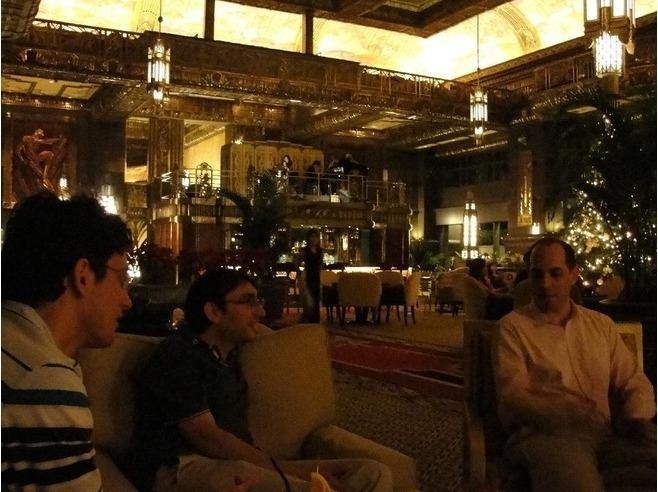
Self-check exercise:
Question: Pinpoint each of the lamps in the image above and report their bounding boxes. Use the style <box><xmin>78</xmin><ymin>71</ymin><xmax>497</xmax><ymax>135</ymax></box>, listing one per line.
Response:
<box><xmin>583</xmin><ymin>0</ymin><xmax>635</xmax><ymax>94</ymax></box>
<box><xmin>469</xmin><ymin>10</ymin><xmax>489</xmax><ymax>146</ymax></box>
<box><xmin>460</xmin><ymin>190</ymin><xmax>480</xmax><ymax>263</ymax></box>
<box><xmin>58</xmin><ymin>161</ymin><xmax>71</xmax><ymax>201</ymax></box>
<box><xmin>99</xmin><ymin>180</ymin><xmax>119</xmax><ymax>198</ymax></box>
<box><xmin>147</xmin><ymin>0</ymin><xmax>172</xmax><ymax>101</ymax></box>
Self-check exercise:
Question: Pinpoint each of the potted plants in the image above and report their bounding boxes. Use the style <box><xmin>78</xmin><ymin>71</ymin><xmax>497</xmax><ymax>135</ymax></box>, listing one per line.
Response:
<box><xmin>489</xmin><ymin>72</ymin><xmax>657</xmax><ymax>386</ymax></box>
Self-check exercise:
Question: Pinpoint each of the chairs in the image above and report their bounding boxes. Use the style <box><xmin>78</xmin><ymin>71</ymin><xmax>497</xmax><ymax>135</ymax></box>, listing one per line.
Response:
<box><xmin>462</xmin><ymin>318</ymin><xmax>644</xmax><ymax>492</ymax></box>
<box><xmin>77</xmin><ymin>327</ymin><xmax>419</xmax><ymax>492</ymax></box>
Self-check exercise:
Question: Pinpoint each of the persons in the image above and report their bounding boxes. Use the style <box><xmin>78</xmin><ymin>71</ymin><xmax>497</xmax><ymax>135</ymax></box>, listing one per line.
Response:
<box><xmin>299</xmin><ymin>228</ymin><xmax>323</xmax><ymax>323</ymax></box>
<box><xmin>1</xmin><ymin>193</ymin><xmax>134</xmax><ymax>492</ymax></box>
<box><xmin>129</xmin><ymin>268</ymin><xmax>394</xmax><ymax>492</ymax></box>
<box><xmin>15</xmin><ymin>127</ymin><xmax>60</xmax><ymax>192</ymax></box>
<box><xmin>470</xmin><ymin>236</ymin><xmax>657</xmax><ymax>492</ymax></box>
<box><xmin>281</xmin><ymin>153</ymin><xmax>362</xmax><ymax>195</ymax></box>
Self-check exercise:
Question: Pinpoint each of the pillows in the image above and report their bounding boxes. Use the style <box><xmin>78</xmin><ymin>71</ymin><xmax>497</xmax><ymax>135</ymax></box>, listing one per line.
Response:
<box><xmin>74</xmin><ymin>330</ymin><xmax>165</xmax><ymax>449</ymax></box>
<box><xmin>239</xmin><ymin>322</ymin><xmax>339</xmax><ymax>460</ymax></box>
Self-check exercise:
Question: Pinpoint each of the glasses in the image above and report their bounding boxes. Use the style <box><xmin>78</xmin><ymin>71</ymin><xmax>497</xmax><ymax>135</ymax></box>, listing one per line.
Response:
<box><xmin>227</xmin><ymin>297</ymin><xmax>267</xmax><ymax>312</ymax></box>
